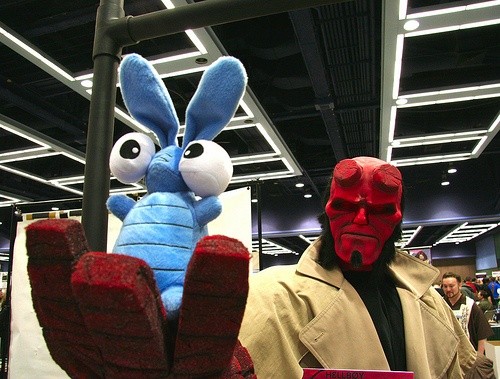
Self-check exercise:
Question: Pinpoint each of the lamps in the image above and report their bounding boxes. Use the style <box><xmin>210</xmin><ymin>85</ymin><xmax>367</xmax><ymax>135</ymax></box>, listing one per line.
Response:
<box><xmin>440</xmin><ymin>162</ymin><xmax>457</xmax><ymax>186</ymax></box>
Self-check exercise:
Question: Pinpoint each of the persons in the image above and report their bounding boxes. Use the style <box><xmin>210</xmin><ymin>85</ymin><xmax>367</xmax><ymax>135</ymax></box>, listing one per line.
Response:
<box><xmin>431</xmin><ymin>271</ymin><xmax>500</xmax><ymax>355</ymax></box>
<box><xmin>237</xmin><ymin>156</ymin><xmax>496</xmax><ymax>379</ymax></box>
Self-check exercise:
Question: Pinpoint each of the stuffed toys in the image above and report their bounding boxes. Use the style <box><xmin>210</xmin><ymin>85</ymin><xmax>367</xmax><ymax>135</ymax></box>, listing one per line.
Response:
<box><xmin>106</xmin><ymin>53</ymin><xmax>248</xmax><ymax>321</ymax></box>
